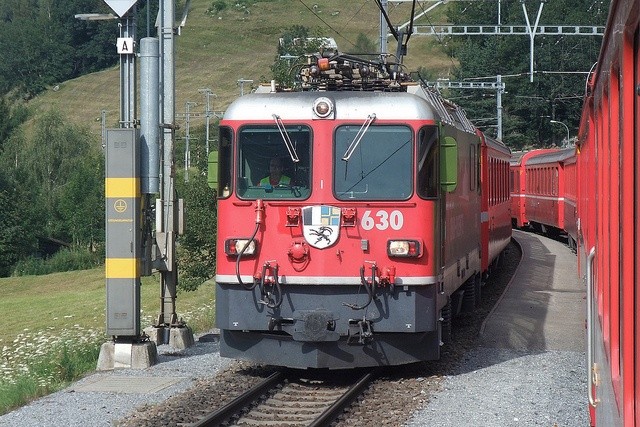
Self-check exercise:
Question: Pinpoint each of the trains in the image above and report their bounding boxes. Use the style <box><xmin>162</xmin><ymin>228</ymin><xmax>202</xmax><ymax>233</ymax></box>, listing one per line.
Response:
<box><xmin>512</xmin><ymin>0</ymin><xmax>640</xmax><ymax>427</ymax></box>
<box><xmin>215</xmin><ymin>44</ymin><xmax>511</xmax><ymax>378</ymax></box>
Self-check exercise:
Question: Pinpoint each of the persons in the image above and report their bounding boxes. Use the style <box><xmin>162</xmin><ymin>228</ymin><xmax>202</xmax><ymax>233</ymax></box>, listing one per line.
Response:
<box><xmin>256</xmin><ymin>153</ymin><xmax>295</xmax><ymax>188</ymax></box>
